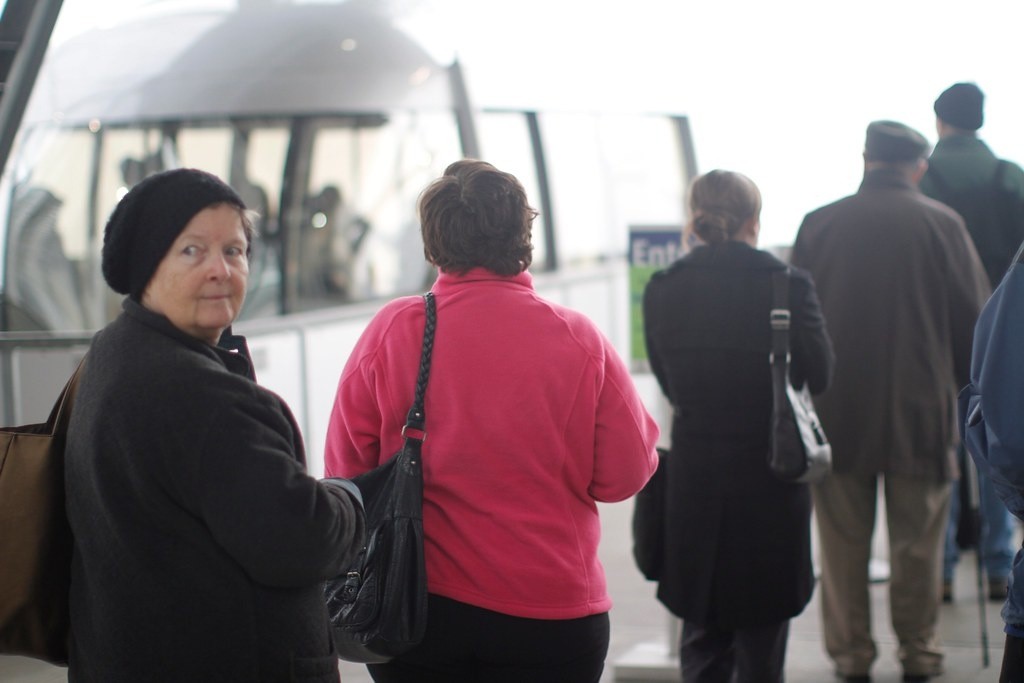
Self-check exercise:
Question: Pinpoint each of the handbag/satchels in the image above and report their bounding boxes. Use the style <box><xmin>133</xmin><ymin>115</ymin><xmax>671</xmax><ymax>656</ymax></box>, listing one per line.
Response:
<box><xmin>321</xmin><ymin>292</ymin><xmax>437</xmax><ymax>664</ymax></box>
<box><xmin>765</xmin><ymin>264</ymin><xmax>832</xmax><ymax>484</ymax></box>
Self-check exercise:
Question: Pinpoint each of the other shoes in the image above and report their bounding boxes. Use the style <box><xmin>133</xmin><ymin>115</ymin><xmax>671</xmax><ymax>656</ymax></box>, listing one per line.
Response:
<box><xmin>834</xmin><ymin>648</ymin><xmax>943</xmax><ymax>682</ymax></box>
<box><xmin>987</xmin><ymin>577</ymin><xmax>1008</xmax><ymax>600</ymax></box>
<box><xmin>943</xmin><ymin>580</ymin><xmax>951</xmax><ymax>602</ymax></box>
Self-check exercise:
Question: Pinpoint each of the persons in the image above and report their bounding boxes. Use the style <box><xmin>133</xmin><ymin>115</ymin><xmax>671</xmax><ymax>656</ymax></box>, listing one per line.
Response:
<box><xmin>642</xmin><ymin>168</ymin><xmax>836</xmax><ymax>683</ymax></box>
<box><xmin>788</xmin><ymin>83</ymin><xmax>1024</xmax><ymax>683</ymax></box>
<box><xmin>324</xmin><ymin>158</ymin><xmax>661</xmax><ymax>683</ymax></box>
<box><xmin>63</xmin><ymin>168</ymin><xmax>366</xmax><ymax>683</ymax></box>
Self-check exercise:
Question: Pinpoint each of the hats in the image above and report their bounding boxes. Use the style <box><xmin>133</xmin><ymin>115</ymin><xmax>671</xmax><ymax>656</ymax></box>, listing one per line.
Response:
<box><xmin>103</xmin><ymin>169</ymin><xmax>247</xmax><ymax>298</ymax></box>
<box><xmin>867</xmin><ymin>122</ymin><xmax>927</xmax><ymax>165</ymax></box>
<box><xmin>935</xmin><ymin>84</ymin><xmax>982</xmax><ymax>130</ymax></box>
